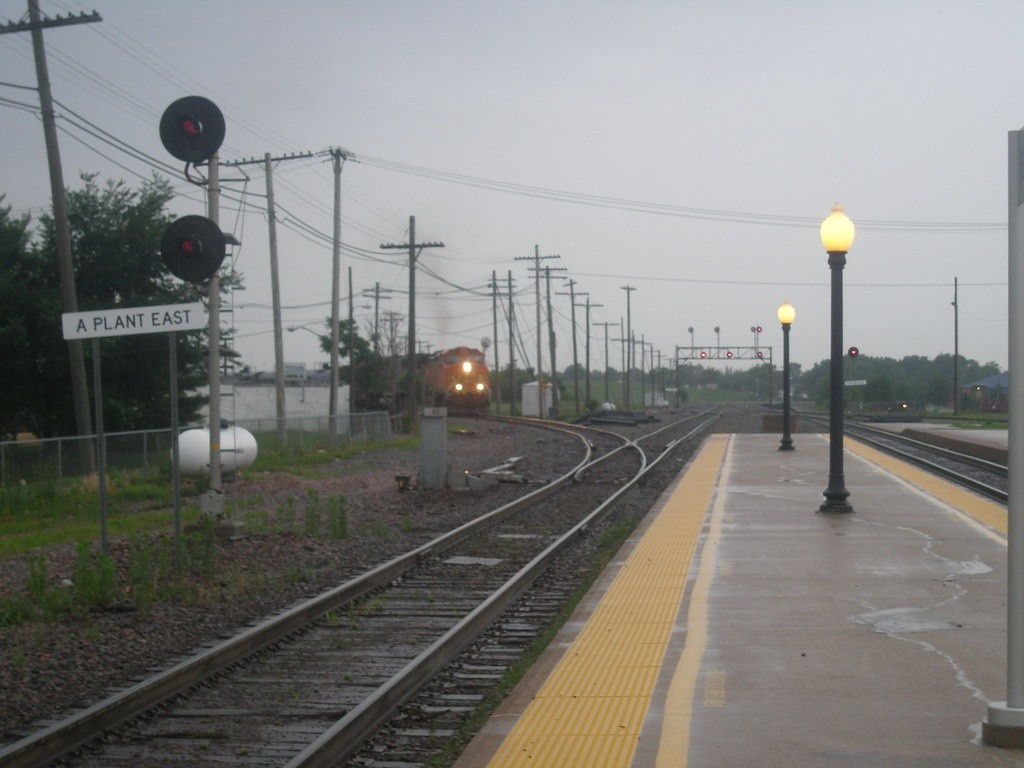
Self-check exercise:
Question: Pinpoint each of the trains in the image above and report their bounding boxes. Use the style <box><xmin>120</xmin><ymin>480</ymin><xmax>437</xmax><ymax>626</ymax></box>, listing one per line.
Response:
<box><xmin>379</xmin><ymin>346</ymin><xmax>490</xmax><ymax>415</ymax></box>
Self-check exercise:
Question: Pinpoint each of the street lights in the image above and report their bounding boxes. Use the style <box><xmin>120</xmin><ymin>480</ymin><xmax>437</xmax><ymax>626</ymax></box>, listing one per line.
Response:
<box><xmin>778</xmin><ymin>299</ymin><xmax>795</xmax><ymax>450</ymax></box>
<box><xmin>820</xmin><ymin>201</ymin><xmax>856</xmax><ymax>511</ymax></box>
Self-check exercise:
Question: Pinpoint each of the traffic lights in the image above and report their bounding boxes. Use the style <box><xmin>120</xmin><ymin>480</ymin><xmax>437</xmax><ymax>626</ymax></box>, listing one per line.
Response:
<box><xmin>159</xmin><ymin>96</ymin><xmax>225</xmax><ymax>163</ymax></box>
<box><xmin>160</xmin><ymin>215</ymin><xmax>225</xmax><ymax>283</ymax></box>
<box><xmin>758</xmin><ymin>352</ymin><xmax>762</xmax><ymax>357</ymax></box>
<box><xmin>848</xmin><ymin>347</ymin><xmax>858</xmax><ymax>357</ymax></box>
<box><xmin>701</xmin><ymin>352</ymin><xmax>706</xmax><ymax>358</ymax></box>
<box><xmin>727</xmin><ymin>352</ymin><xmax>732</xmax><ymax>357</ymax></box>
<box><xmin>757</xmin><ymin>326</ymin><xmax>762</xmax><ymax>332</ymax></box>
